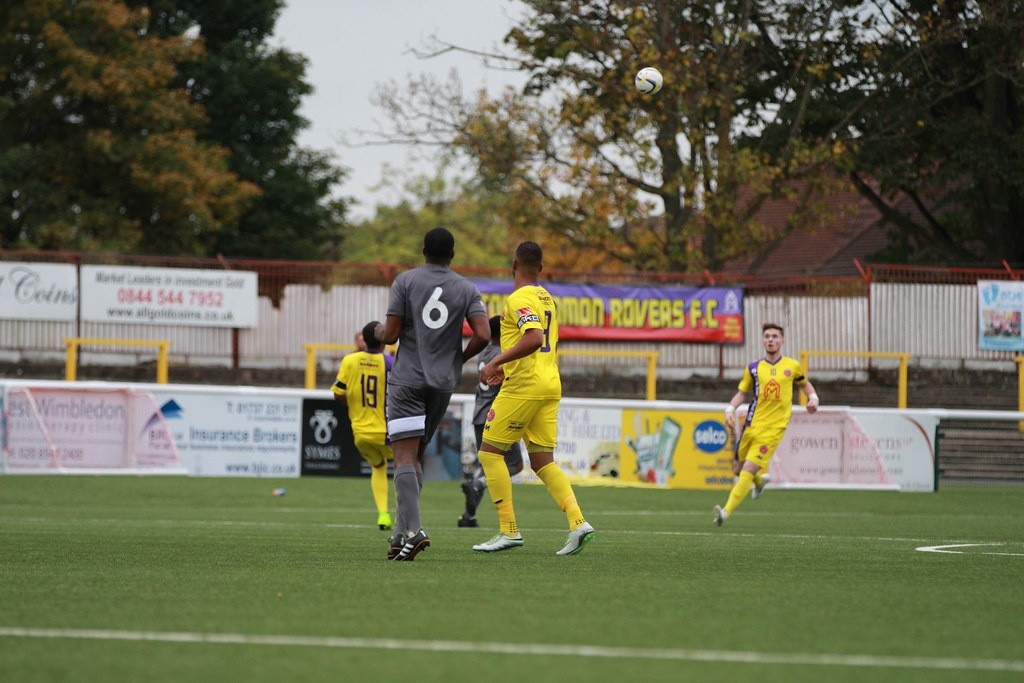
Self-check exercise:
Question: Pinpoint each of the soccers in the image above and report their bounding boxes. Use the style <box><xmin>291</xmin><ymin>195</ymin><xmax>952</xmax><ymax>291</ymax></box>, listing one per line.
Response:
<box><xmin>636</xmin><ymin>66</ymin><xmax>663</xmax><ymax>95</ymax></box>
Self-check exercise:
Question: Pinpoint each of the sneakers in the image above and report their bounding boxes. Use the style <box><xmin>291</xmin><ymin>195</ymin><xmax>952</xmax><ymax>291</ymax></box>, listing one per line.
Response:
<box><xmin>471</xmin><ymin>531</ymin><xmax>525</xmax><ymax>554</ymax></box>
<box><xmin>555</xmin><ymin>522</ymin><xmax>596</xmax><ymax>556</ymax></box>
<box><xmin>460</xmin><ymin>481</ymin><xmax>479</xmax><ymax>516</ymax></box>
<box><xmin>712</xmin><ymin>504</ymin><xmax>725</xmax><ymax>527</ymax></box>
<box><xmin>751</xmin><ymin>473</ymin><xmax>772</xmax><ymax>501</ymax></box>
<box><xmin>386</xmin><ymin>533</ymin><xmax>407</xmax><ymax>560</ymax></box>
<box><xmin>458</xmin><ymin>518</ymin><xmax>480</xmax><ymax>528</ymax></box>
<box><xmin>378</xmin><ymin>513</ymin><xmax>393</xmax><ymax>530</ymax></box>
<box><xmin>392</xmin><ymin>527</ymin><xmax>431</xmax><ymax>562</ymax></box>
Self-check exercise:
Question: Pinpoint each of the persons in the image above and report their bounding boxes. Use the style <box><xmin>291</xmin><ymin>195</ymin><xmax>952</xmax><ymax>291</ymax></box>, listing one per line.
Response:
<box><xmin>331</xmin><ymin>320</ymin><xmax>394</xmax><ymax>531</ymax></box>
<box><xmin>472</xmin><ymin>240</ymin><xmax>596</xmax><ymax>556</ymax></box>
<box><xmin>458</xmin><ymin>314</ymin><xmax>523</xmax><ymax>528</ymax></box>
<box><xmin>373</xmin><ymin>228</ymin><xmax>491</xmax><ymax>562</ymax></box>
<box><xmin>714</xmin><ymin>323</ymin><xmax>819</xmax><ymax>526</ymax></box>
<box><xmin>354</xmin><ymin>333</ymin><xmax>367</xmax><ymax>351</ymax></box>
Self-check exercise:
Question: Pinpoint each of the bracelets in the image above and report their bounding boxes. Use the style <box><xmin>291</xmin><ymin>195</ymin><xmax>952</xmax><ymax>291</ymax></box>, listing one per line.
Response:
<box><xmin>809</xmin><ymin>393</ymin><xmax>819</xmax><ymax>400</ymax></box>
<box><xmin>725</xmin><ymin>404</ymin><xmax>735</xmax><ymax>414</ymax></box>
